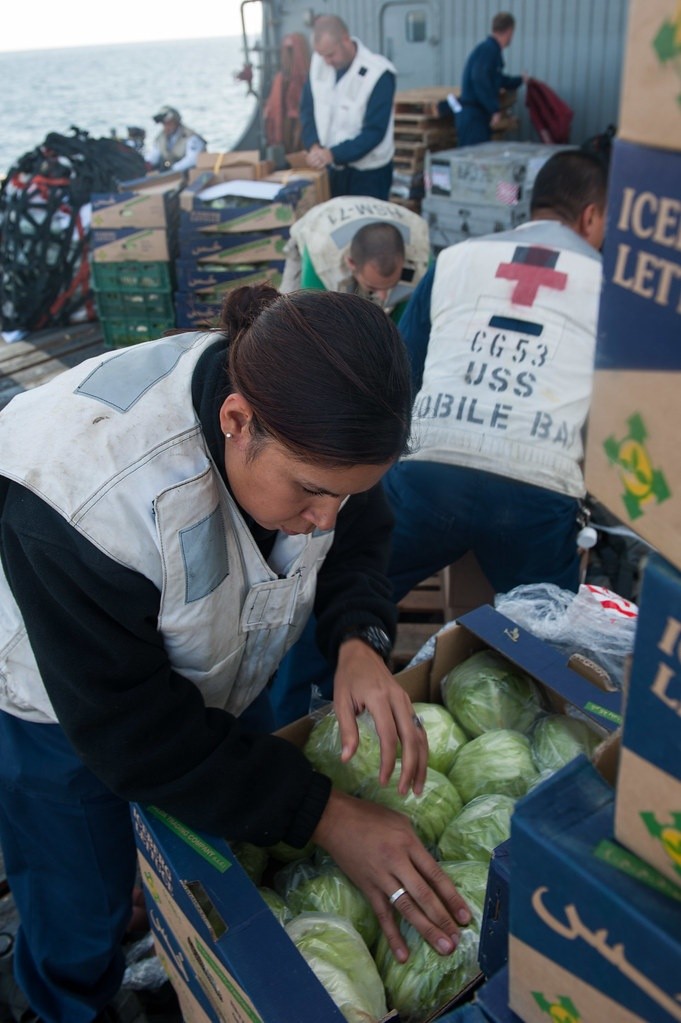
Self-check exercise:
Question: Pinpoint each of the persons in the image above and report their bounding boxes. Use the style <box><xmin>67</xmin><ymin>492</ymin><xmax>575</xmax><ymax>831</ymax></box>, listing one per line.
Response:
<box><xmin>0</xmin><ymin>286</ymin><xmax>476</xmax><ymax>1023</ymax></box>
<box><xmin>125</xmin><ymin>13</ymin><xmax>608</xmax><ymax>700</ymax></box>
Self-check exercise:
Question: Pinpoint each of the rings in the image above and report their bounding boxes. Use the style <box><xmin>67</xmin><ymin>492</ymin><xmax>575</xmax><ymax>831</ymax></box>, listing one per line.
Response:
<box><xmin>386</xmin><ymin>885</ymin><xmax>406</xmax><ymax>904</ymax></box>
<box><xmin>412</xmin><ymin>714</ymin><xmax>422</xmax><ymax>725</ymax></box>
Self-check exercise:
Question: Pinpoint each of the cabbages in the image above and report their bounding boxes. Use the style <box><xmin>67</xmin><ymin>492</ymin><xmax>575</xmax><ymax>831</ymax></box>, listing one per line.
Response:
<box><xmin>189</xmin><ymin>648</ymin><xmax>612</xmax><ymax>1023</ymax></box>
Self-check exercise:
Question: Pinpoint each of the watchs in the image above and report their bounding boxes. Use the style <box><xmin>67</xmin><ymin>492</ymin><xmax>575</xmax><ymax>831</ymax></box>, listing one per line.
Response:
<box><xmin>347</xmin><ymin>618</ymin><xmax>391</xmax><ymax>657</ymax></box>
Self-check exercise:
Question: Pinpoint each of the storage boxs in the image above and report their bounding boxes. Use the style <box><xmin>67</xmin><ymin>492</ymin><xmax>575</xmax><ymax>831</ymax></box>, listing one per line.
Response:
<box><xmin>0</xmin><ymin>0</ymin><xmax>681</xmax><ymax>1023</ymax></box>
